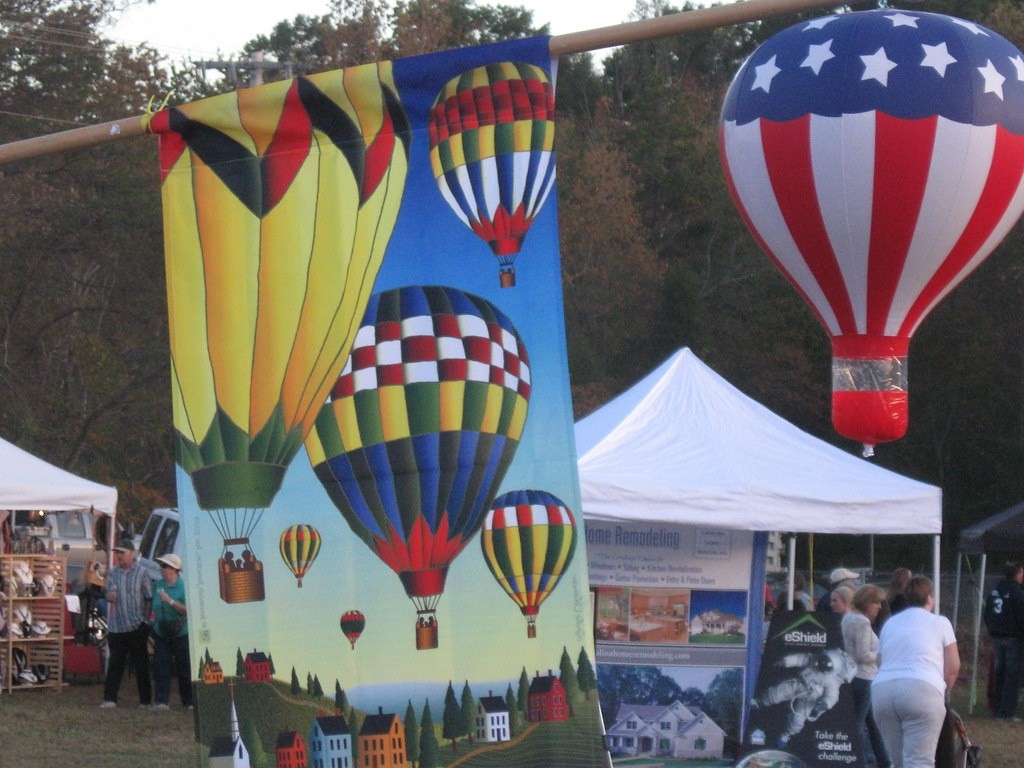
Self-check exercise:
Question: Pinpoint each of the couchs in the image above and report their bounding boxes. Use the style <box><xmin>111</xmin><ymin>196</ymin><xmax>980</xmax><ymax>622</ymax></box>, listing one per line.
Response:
<box><xmin>35</xmin><ymin>582</ymin><xmax>102</xmax><ymax>679</ymax></box>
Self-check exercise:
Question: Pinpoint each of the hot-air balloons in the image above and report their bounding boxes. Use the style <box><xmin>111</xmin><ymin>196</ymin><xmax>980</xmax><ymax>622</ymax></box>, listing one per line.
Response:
<box><xmin>279</xmin><ymin>524</ymin><xmax>322</xmax><ymax>588</ymax></box>
<box><xmin>340</xmin><ymin>610</ymin><xmax>366</xmax><ymax>650</ymax></box>
<box><xmin>715</xmin><ymin>7</ymin><xmax>1024</xmax><ymax>461</ymax></box>
<box><xmin>425</xmin><ymin>60</ymin><xmax>560</xmax><ymax>287</ymax></box>
<box><xmin>480</xmin><ymin>489</ymin><xmax>578</xmax><ymax>638</ymax></box>
<box><xmin>159</xmin><ymin>62</ymin><xmax>413</xmax><ymax>605</ymax></box>
<box><xmin>302</xmin><ymin>284</ymin><xmax>533</xmax><ymax>652</ymax></box>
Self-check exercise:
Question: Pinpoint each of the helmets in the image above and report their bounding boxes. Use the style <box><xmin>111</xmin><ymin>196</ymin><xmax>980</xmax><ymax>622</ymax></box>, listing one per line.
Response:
<box><xmin>814</xmin><ymin>651</ymin><xmax>842</xmax><ymax>677</ymax></box>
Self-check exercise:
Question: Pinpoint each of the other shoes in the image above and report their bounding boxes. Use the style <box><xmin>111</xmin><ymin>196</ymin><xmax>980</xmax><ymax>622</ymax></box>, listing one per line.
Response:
<box><xmin>997</xmin><ymin>716</ymin><xmax>1022</xmax><ymax>723</ymax></box>
<box><xmin>99</xmin><ymin>700</ymin><xmax>122</xmax><ymax>710</ymax></box>
<box><xmin>137</xmin><ymin>703</ymin><xmax>171</xmax><ymax>711</ymax></box>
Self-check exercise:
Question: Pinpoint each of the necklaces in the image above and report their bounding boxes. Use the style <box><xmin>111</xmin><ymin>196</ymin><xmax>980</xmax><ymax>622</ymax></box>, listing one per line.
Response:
<box><xmin>18</xmin><ymin>567</ymin><xmax>31</xmax><ymax>576</ymax></box>
<box><xmin>19</xmin><ymin>609</ymin><xmax>28</xmax><ymax>621</ymax></box>
<box><xmin>35</xmin><ymin>623</ymin><xmax>48</xmax><ymax>631</ymax></box>
<box><xmin>43</xmin><ymin>578</ymin><xmax>56</xmax><ymax>592</ymax></box>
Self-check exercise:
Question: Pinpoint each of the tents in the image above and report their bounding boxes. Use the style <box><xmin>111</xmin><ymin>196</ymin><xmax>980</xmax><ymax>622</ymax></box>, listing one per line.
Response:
<box><xmin>573</xmin><ymin>345</ymin><xmax>944</xmax><ymax>618</ymax></box>
<box><xmin>952</xmin><ymin>501</ymin><xmax>1024</xmax><ymax>715</ymax></box>
<box><xmin>1</xmin><ymin>435</ymin><xmax>117</xmax><ymax>571</ymax></box>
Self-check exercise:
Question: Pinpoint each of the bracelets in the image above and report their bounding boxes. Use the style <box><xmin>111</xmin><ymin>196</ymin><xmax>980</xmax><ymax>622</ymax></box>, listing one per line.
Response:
<box><xmin>170</xmin><ymin>599</ymin><xmax>174</xmax><ymax>607</ymax></box>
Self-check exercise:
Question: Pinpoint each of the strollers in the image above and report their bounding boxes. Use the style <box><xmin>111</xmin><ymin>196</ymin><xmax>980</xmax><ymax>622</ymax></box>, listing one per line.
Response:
<box><xmin>936</xmin><ymin>697</ymin><xmax>982</xmax><ymax>768</ymax></box>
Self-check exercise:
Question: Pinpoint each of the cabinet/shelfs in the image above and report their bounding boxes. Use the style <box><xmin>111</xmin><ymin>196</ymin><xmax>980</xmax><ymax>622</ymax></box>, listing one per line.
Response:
<box><xmin>1</xmin><ymin>554</ymin><xmax>75</xmax><ymax>696</ymax></box>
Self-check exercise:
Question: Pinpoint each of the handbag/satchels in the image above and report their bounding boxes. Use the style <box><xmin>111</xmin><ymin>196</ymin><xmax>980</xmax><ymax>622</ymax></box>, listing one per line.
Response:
<box><xmin>157</xmin><ymin>618</ymin><xmax>183</xmax><ymax>638</ymax></box>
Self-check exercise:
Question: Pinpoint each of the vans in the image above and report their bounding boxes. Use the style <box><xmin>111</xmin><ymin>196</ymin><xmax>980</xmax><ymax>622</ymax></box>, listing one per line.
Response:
<box><xmin>0</xmin><ymin>503</ymin><xmax>144</xmax><ymax>583</ymax></box>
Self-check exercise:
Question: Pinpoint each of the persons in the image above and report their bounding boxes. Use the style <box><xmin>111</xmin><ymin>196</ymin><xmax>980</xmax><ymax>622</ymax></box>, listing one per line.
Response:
<box><xmin>765</xmin><ymin>567</ymin><xmax>913</xmax><ymax>768</ymax></box>
<box><xmin>146</xmin><ymin>553</ymin><xmax>194</xmax><ymax>712</ymax></box>
<box><xmin>983</xmin><ymin>560</ymin><xmax>1024</xmax><ymax>723</ymax></box>
<box><xmin>869</xmin><ymin>573</ymin><xmax>961</xmax><ymax>768</ymax></box>
<box><xmin>72</xmin><ymin>584</ymin><xmax>110</xmax><ymax>679</ymax></box>
<box><xmin>98</xmin><ymin>538</ymin><xmax>154</xmax><ymax>710</ymax></box>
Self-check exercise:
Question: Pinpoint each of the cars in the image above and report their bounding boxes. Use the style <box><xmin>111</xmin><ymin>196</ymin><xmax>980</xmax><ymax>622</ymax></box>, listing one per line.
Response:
<box><xmin>136</xmin><ymin>507</ymin><xmax>184</xmax><ymax>587</ymax></box>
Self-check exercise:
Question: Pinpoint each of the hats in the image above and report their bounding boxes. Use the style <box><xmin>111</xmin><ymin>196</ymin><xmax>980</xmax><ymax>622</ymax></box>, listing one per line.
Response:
<box><xmin>828</xmin><ymin>567</ymin><xmax>857</xmax><ymax>584</ymax></box>
<box><xmin>155</xmin><ymin>553</ymin><xmax>181</xmax><ymax>570</ymax></box>
<box><xmin>111</xmin><ymin>538</ymin><xmax>135</xmax><ymax>550</ymax></box>
